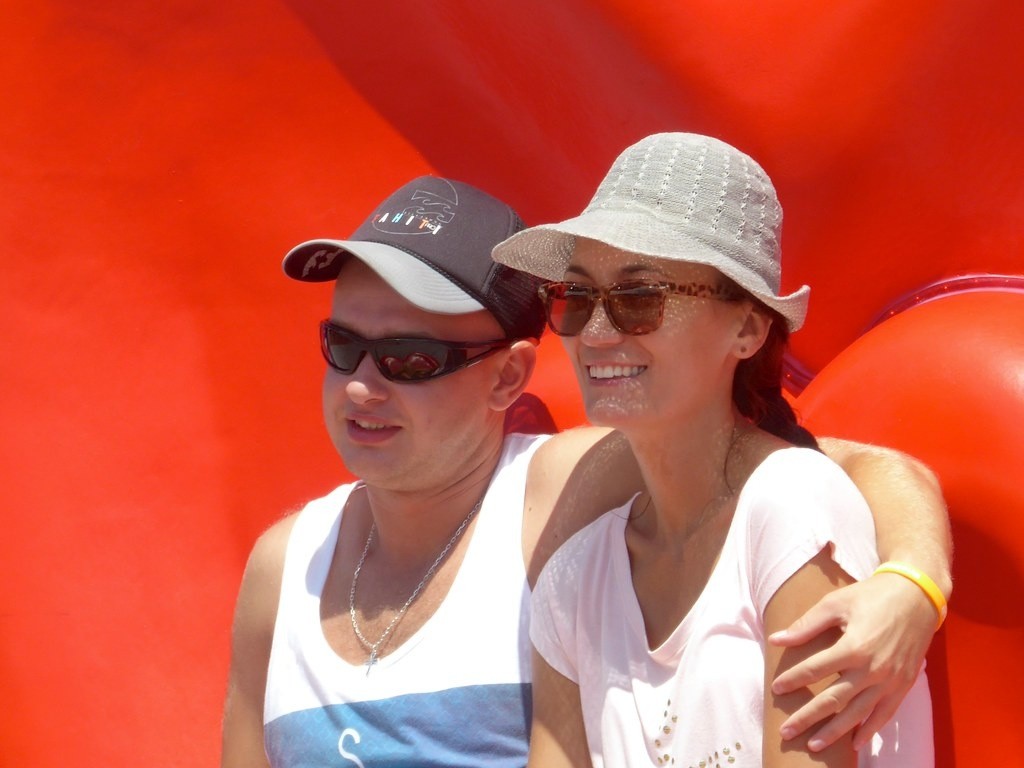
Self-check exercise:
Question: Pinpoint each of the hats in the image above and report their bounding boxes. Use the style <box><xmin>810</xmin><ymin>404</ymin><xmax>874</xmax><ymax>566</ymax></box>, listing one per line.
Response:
<box><xmin>490</xmin><ymin>132</ymin><xmax>810</xmax><ymax>333</ymax></box>
<box><xmin>281</xmin><ymin>176</ymin><xmax>548</xmax><ymax>339</ymax></box>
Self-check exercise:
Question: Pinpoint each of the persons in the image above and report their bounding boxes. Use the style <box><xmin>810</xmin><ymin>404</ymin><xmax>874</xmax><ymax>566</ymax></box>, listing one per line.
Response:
<box><xmin>489</xmin><ymin>131</ymin><xmax>934</xmax><ymax>768</ymax></box>
<box><xmin>221</xmin><ymin>175</ymin><xmax>955</xmax><ymax>768</ymax></box>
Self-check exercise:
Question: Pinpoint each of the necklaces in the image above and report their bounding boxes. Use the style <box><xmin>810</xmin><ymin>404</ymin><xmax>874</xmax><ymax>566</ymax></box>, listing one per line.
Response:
<box><xmin>349</xmin><ymin>499</ymin><xmax>482</xmax><ymax>676</ymax></box>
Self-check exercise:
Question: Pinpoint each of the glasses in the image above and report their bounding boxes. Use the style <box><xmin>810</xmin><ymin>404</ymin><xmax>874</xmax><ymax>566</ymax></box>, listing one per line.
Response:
<box><xmin>536</xmin><ymin>277</ymin><xmax>757</xmax><ymax>337</ymax></box>
<box><xmin>318</xmin><ymin>320</ymin><xmax>540</xmax><ymax>384</ymax></box>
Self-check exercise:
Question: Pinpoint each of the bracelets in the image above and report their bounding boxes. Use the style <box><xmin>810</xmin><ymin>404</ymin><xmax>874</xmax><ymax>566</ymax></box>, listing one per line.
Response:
<box><xmin>876</xmin><ymin>560</ymin><xmax>947</xmax><ymax>632</ymax></box>
